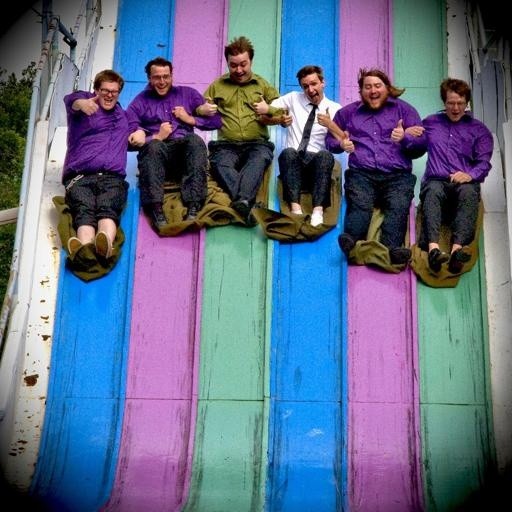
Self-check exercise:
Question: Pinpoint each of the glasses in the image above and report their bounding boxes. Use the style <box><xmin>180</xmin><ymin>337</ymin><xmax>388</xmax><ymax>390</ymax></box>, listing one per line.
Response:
<box><xmin>100</xmin><ymin>89</ymin><xmax>119</xmax><ymax>95</ymax></box>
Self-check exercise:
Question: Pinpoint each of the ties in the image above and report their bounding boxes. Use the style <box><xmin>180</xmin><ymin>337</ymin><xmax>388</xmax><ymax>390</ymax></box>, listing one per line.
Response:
<box><xmin>298</xmin><ymin>104</ymin><xmax>318</xmax><ymax>152</ymax></box>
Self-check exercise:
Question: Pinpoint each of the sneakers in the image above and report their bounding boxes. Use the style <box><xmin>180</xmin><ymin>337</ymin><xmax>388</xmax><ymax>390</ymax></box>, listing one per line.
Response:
<box><xmin>389</xmin><ymin>247</ymin><xmax>412</xmax><ymax>263</ymax></box>
<box><xmin>428</xmin><ymin>248</ymin><xmax>449</xmax><ymax>272</ymax></box>
<box><xmin>449</xmin><ymin>246</ymin><xmax>472</xmax><ymax>274</ymax></box>
<box><xmin>95</xmin><ymin>231</ymin><xmax>110</xmax><ymax>259</ymax></box>
<box><xmin>338</xmin><ymin>234</ymin><xmax>356</xmax><ymax>255</ymax></box>
<box><xmin>150</xmin><ymin>208</ymin><xmax>198</xmax><ymax>231</ymax></box>
<box><xmin>310</xmin><ymin>214</ymin><xmax>323</xmax><ymax>227</ymax></box>
<box><xmin>228</xmin><ymin>200</ymin><xmax>251</xmax><ymax>218</ymax></box>
<box><xmin>67</xmin><ymin>237</ymin><xmax>82</xmax><ymax>254</ymax></box>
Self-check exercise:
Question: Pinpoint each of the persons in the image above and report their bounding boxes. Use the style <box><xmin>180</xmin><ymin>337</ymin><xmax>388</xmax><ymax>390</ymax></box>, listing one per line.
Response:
<box><xmin>62</xmin><ymin>70</ymin><xmax>146</xmax><ymax>268</ymax></box>
<box><xmin>325</xmin><ymin>69</ymin><xmax>427</xmax><ymax>264</ymax></box>
<box><xmin>195</xmin><ymin>37</ymin><xmax>287</xmax><ymax>223</ymax></box>
<box><xmin>125</xmin><ymin>57</ymin><xmax>224</xmax><ymax>233</ymax></box>
<box><xmin>260</xmin><ymin>65</ymin><xmax>349</xmax><ymax>227</ymax></box>
<box><xmin>405</xmin><ymin>78</ymin><xmax>494</xmax><ymax>274</ymax></box>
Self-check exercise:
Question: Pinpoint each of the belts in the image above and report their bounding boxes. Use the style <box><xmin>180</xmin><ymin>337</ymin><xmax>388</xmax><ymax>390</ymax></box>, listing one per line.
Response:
<box><xmin>80</xmin><ymin>171</ymin><xmax>118</xmax><ymax>175</ymax></box>
